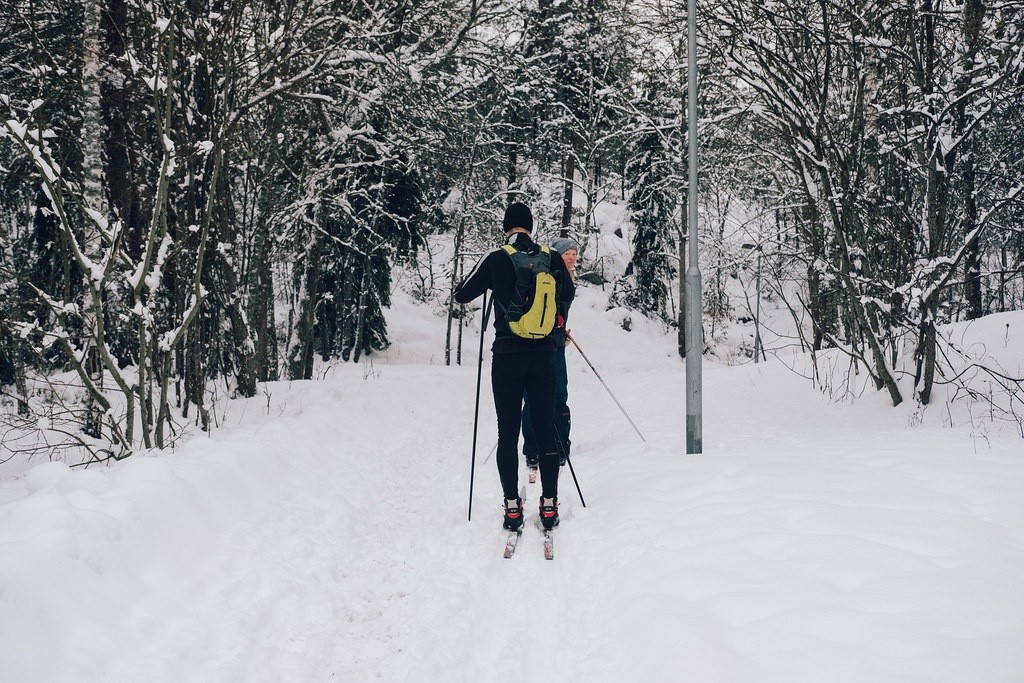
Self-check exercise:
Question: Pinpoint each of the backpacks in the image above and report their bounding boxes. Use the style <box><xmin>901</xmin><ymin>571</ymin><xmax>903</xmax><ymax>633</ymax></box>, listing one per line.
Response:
<box><xmin>483</xmin><ymin>244</ymin><xmax>558</xmax><ymax>339</ymax></box>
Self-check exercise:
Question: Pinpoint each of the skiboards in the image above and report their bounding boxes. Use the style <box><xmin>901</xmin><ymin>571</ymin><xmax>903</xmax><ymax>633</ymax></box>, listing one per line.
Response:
<box><xmin>504</xmin><ymin>485</ymin><xmax>555</xmax><ymax>561</ymax></box>
<box><xmin>529</xmin><ymin>472</ymin><xmax>537</xmax><ymax>484</ymax></box>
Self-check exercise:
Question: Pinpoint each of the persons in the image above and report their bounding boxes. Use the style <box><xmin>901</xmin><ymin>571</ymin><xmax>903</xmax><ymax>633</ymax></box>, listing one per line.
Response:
<box><xmin>522</xmin><ymin>239</ymin><xmax>578</xmax><ymax>467</ymax></box>
<box><xmin>453</xmin><ymin>202</ymin><xmax>575</xmax><ymax>533</ymax></box>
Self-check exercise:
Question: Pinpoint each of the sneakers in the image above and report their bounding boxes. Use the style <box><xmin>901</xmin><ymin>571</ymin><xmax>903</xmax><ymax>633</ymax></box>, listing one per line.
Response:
<box><xmin>540</xmin><ymin>496</ymin><xmax>559</xmax><ymax>528</ymax></box>
<box><xmin>527</xmin><ymin>454</ymin><xmax>539</xmax><ymax>466</ymax></box>
<box><xmin>502</xmin><ymin>497</ymin><xmax>523</xmax><ymax>527</ymax></box>
<box><xmin>560</xmin><ymin>455</ymin><xmax>567</xmax><ymax>465</ymax></box>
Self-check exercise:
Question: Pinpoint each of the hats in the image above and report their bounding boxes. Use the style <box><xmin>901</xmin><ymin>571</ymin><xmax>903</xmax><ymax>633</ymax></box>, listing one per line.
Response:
<box><xmin>503</xmin><ymin>202</ymin><xmax>533</xmax><ymax>232</ymax></box>
<box><xmin>552</xmin><ymin>238</ymin><xmax>578</xmax><ymax>255</ymax></box>
